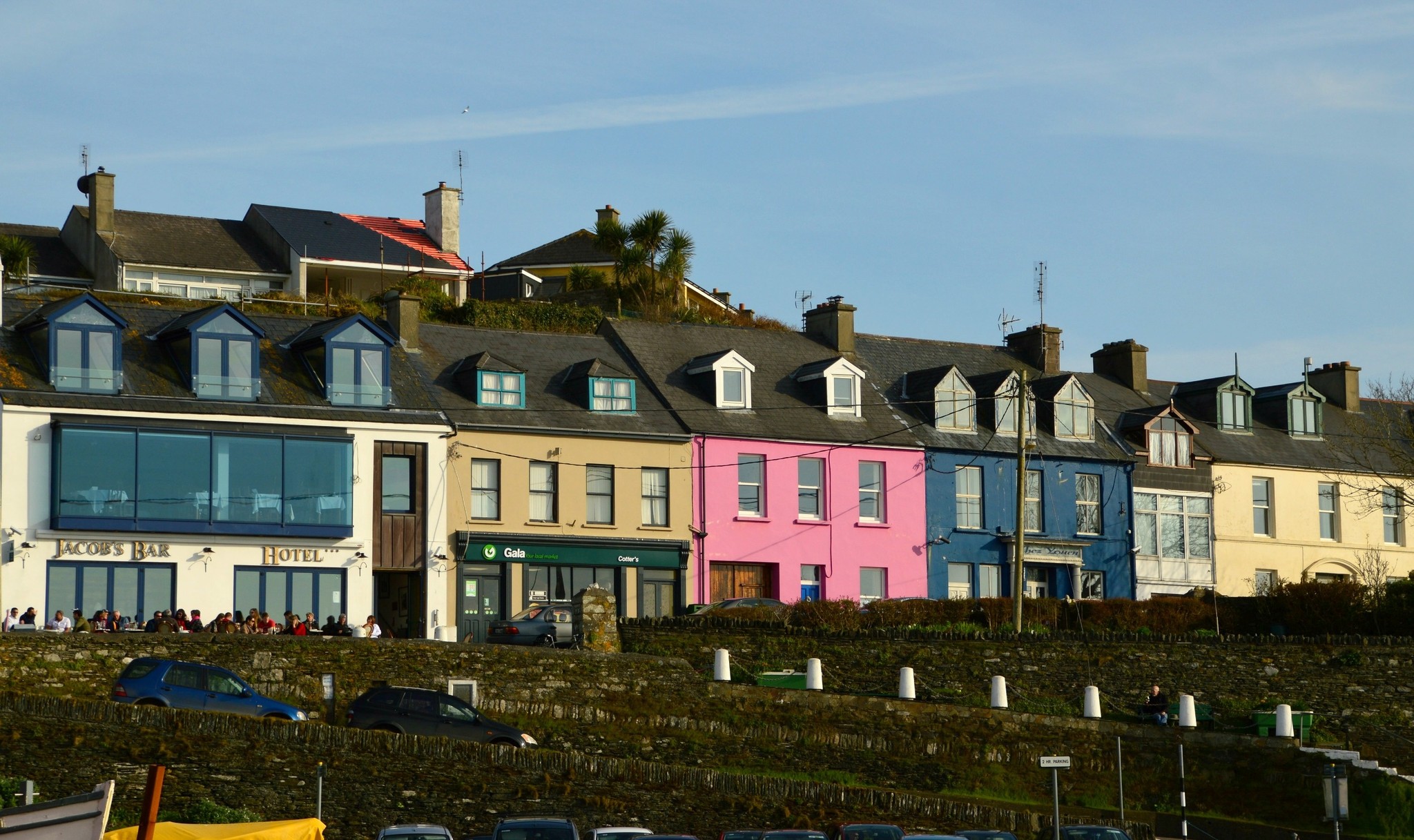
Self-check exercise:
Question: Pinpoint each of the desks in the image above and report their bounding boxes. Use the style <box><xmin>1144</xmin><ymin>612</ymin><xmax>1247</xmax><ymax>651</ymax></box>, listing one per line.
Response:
<box><xmin>69</xmin><ymin>489</ymin><xmax>129</xmax><ymax>515</ymax></box>
<box><xmin>38</xmin><ymin>629</ymin><xmax>59</xmax><ymax>632</ymax></box>
<box><xmin>97</xmin><ymin>628</ymin><xmax>145</xmax><ymax>633</ymax></box>
<box><xmin>184</xmin><ymin>491</ymin><xmax>223</xmax><ymax>520</ymax></box>
<box><xmin>305</xmin><ymin>497</ymin><xmax>346</xmax><ymax>524</ymax></box>
<box><xmin>310</xmin><ymin>629</ymin><xmax>324</xmax><ymax>636</ymax></box>
<box><xmin>247</xmin><ymin>492</ymin><xmax>280</xmax><ymax>521</ymax></box>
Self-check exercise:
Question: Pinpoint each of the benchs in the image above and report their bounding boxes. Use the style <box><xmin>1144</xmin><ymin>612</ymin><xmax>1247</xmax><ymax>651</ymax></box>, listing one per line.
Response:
<box><xmin>1165</xmin><ymin>703</ymin><xmax>1216</xmax><ymax>730</ymax></box>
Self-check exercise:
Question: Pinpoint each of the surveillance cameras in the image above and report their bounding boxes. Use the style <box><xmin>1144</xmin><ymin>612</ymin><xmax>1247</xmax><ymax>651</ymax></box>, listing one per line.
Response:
<box><xmin>10</xmin><ymin>526</ymin><xmax>23</xmax><ymax>535</ymax></box>
<box><xmin>434</xmin><ymin>547</ymin><xmax>443</xmax><ymax>557</ymax></box>
<box><xmin>1131</xmin><ymin>545</ymin><xmax>1142</xmax><ymax>554</ymax></box>
<box><xmin>938</xmin><ymin>536</ymin><xmax>951</xmax><ymax>544</ymax></box>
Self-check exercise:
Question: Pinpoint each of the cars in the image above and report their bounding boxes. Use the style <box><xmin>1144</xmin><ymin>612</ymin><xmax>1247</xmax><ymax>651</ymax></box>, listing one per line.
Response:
<box><xmin>110</xmin><ymin>656</ymin><xmax>310</xmax><ymax>722</ymax></box>
<box><xmin>1035</xmin><ymin>824</ymin><xmax>1133</xmax><ymax>840</ymax></box>
<box><xmin>344</xmin><ymin>684</ymin><xmax>540</xmax><ymax>750</ymax></box>
<box><xmin>858</xmin><ymin>597</ymin><xmax>939</xmax><ymax>616</ymax></box>
<box><xmin>375</xmin><ymin>823</ymin><xmax>454</xmax><ymax>840</ymax></box>
<box><xmin>583</xmin><ymin>825</ymin><xmax>1017</xmax><ymax>840</ymax></box>
<box><xmin>683</xmin><ymin>596</ymin><xmax>803</xmax><ymax>620</ymax></box>
<box><xmin>485</xmin><ymin>604</ymin><xmax>573</xmax><ymax>646</ymax></box>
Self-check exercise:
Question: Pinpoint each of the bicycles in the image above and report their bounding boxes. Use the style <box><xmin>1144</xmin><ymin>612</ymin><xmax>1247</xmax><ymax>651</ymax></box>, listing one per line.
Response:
<box><xmin>534</xmin><ymin>631</ymin><xmax>593</xmax><ymax>652</ymax></box>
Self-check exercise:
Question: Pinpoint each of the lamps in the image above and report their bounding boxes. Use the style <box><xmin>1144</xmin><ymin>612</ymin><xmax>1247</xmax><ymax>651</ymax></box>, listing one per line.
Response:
<box><xmin>20</xmin><ymin>542</ymin><xmax>34</xmax><ymax>549</ymax></box>
<box><xmin>440</xmin><ymin>554</ymin><xmax>449</xmax><ymax>560</ymax></box>
<box><xmin>356</xmin><ymin>551</ymin><xmax>368</xmax><ymax>559</ymax></box>
<box><xmin>199</xmin><ymin>547</ymin><xmax>215</xmax><ymax>555</ymax></box>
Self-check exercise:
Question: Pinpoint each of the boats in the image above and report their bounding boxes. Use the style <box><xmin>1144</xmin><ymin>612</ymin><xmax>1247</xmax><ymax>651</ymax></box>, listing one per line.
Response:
<box><xmin>0</xmin><ymin>778</ymin><xmax>116</xmax><ymax>840</ymax></box>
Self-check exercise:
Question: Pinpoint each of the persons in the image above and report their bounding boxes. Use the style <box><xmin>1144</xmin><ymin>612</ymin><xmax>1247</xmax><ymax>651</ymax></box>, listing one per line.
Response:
<box><xmin>88</xmin><ymin>610</ymin><xmax>101</xmax><ymax>623</ymax></box>
<box><xmin>211</xmin><ymin>677</ymin><xmax>231</xmax><ymax>694</ymax></box>
<box><xmin>302</xmin><ymin>612</ymin><xmax>319</xmax><ymax>630</ymax></box>
<box><xmin>208</xmin><ymin>613</ymin><xmax>225</xmax><ymax>632</ymax></box>
<box><xmin>109</xmin><ymin>610</ymin><xmax>129</xmax><ymax>632</ymax></box>
<box><xmin>217</xmin><ymin>612</ymin><xmax>236</xmax><ymax>633</ymax></box>
<box><xmin>1143</xmin><ymin>686</ymin><xmax>1169</xmax><ymax>726</ymax></box>
<box><xmin>364</xmin><ymin>615</ymin><xmax>382</xmax><ymax>638</ymax></box>
<box><xmin>333</xmin><ymin>613</ymin><xmax>348</xmax><ymax>636</ymax></box>
<box><xmin>235</xmin><ymin>611</ymin><xmax>244</xmax><ymax>626</ymax></box>
<box><xmin>19</xmin><ymin>607</ymin><xmax>36</xmax><ymax>625</ymax></box>
<box><xmin>3</xmin><ymin>607</ymin><xmax>19</xmax><ymax>632</ymax></box>
<box><xmin>849</xmin><ymin>831</ymin><xmax>859</xmax><ymax>840</ymax></box>
<box><xmin>93</xmin><ymin>609</ymin><xmax>111</xmax><ymax>633</ymax></box>
<box><xmin>241</xmin><ymin>608</ymin><xmax>275</xmax><ymax>635</ymax></box>
<box><xmin>320</xmin><ymin>616</ymin><xmax>336</xmax><ymax>635</ymax></box>
<box><xmin>281</xmin><ymin>611</ymin><xmax>311</xmax><ymax>635</ymax></box>
<box><xmin>145</xmin><ymin>609</ymin><xmax>187</xmax><ymax>633</ymax></box>
<box><xmin>46</xmin><ymin>610</ymin><xmax>71</xmax><ymax>632</ymax></box>
<box><xmin>72</xmin><ymin>610</ymin><xmax>91</xmax><ymax>633</ymax></box>
<box><xmin>184</xmin><ymin>610</ymin><xmax>203</xmax><ymax>632</ymax></box>
<box><xmin>125</xmin><ymin>615</ymin><xmax>138</xmax><ymax>624</ymax></box>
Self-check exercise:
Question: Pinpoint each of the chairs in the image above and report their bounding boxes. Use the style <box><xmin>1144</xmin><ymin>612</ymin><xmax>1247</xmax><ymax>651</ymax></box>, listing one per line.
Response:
<box><xmin>275</xmin><ymin>623</ymin><xmax>283</xmax><ymax>631</ymax></box>
<box><xmin>559</xmin><ymin>613</ymin><xmax>567</xmax><ymax>621</ymax></box>
<box><xmin>463</xmin><ymin>631</ymin><xmax>473</xmax><ymax>642</ymax></box>
<box><xmin>88</xmin><ymin>620</ymin><xmax>96</xmax><ymax>632</ymax></box>
<box><xmin>105</xmin><ymin>484</ymin><xmax>124</xmax><ymax>517</ymax></box>
<box><xmin>387</xmin><ymin>629</ymin><xmax>393</xmax><ymax>638</ymax></box>
<box><xmin>226</xmin><ymin>620</ymin><xmax>238</xmax><ymax>633</ymax></box>
<box><xmin>157</xmin><ymin>621</ymin><xmax>173</xmax><ymax>633</ymax></box>
<box><xmin>363</xmin><ymin>623</ymin><xmax>374</xmax><ymax>638</ymax></box>
<box><xmin>205</xmin><ymin>624</ymin><xmax>212</xmax><ymax>632</ymax></box>
<box><xmin>231</xmin><ymin>487</ymin><xmax>251</xmax><ymax>521</ymax></box>
<box><xmin>217</xmin><ymin>620</ymin><xmax>227</xmax><ymax>633</ymax></box>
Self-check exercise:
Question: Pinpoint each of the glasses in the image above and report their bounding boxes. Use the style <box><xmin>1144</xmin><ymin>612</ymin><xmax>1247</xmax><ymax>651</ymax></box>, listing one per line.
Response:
<box><xmin>177</xmin><ymin>613</ymin><xmax>182</xmax><ymax>615</ymax></box>
<box><xmin>250</xmin><ymin>620</ymin><xmax>255</xmax><ymax>623</ymax></box>
<box><xmin>251</xmin><ymin>612</ymin><xmax>254</xmax><ymax>613</ymax></box>
<box><xmin>156</xmin><ymin>615</ymin><xmax>162</xmax><ymax>617</ymax></box>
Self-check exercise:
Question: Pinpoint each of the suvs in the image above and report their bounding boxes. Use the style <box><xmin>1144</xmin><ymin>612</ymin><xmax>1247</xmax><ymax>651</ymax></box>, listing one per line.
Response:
<box><xmin>491</xmin><ymin>816</ymin><xmax>579</xmax><ymax>840</ymax></box>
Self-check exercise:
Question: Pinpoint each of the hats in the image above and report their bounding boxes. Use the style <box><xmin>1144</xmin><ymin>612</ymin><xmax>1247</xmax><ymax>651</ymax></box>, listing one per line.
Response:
<box><xmin>102</xmin><ymin>609</ymin><xmax>111</xmax><ymax>615</ymax></box>
